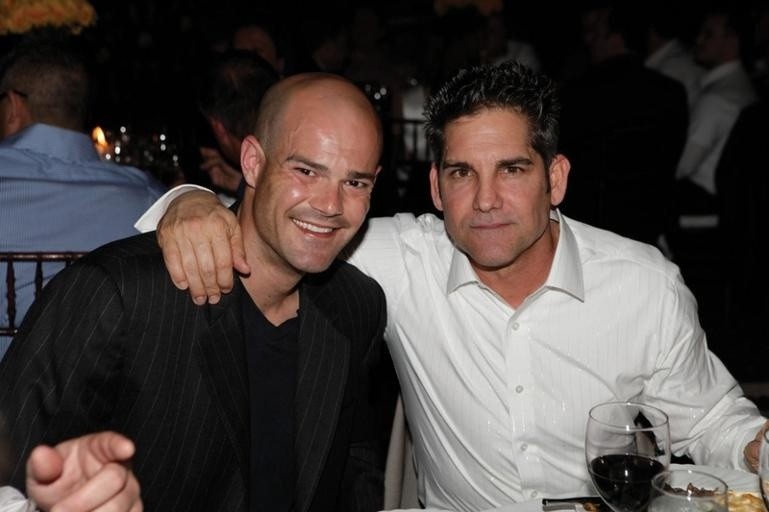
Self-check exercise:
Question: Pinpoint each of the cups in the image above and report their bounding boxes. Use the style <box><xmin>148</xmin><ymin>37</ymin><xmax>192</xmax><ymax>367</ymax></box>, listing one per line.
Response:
<box><xmin>584</xmin><ymin>403</ymin><xmax>728</xmax><ymax>512</ymax></box>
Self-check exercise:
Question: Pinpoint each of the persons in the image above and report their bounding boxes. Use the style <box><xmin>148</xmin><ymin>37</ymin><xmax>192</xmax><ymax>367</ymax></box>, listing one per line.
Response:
<box><xmin>134</xmin><ymin>59</ymin><xmax>768</xmax><ymax>512</ymax></box>
<box><xmin>1</xmin><ymin>0</ymin><xmax>769</xmax><ymax>360</ymax></box>
<box><xmin>1</xmin><ymin>69</ymin><xmax>401</xmax><ymax>512</ymax></box>
